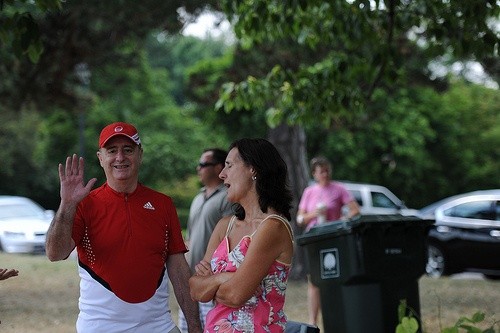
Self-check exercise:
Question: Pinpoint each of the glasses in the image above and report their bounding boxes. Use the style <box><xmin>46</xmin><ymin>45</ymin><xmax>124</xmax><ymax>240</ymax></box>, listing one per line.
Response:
<box><xmin>199</xmin><ymin>163</ymin><xmax>222</xmax><ymax>168</ymax></box>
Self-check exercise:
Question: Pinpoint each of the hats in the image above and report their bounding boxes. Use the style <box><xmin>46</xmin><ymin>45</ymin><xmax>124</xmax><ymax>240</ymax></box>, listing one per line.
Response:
<box><xmin>98</xmin><ymin>123</ymin><xmax>141</xmax><ymax>149</ymax></box>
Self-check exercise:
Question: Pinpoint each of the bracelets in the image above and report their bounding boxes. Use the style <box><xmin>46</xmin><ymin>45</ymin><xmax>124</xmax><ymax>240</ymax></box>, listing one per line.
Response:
<box><xmin>345</xmin><ymin>215</ymin><xmax>349</xmax><ymax>219</ymax></box>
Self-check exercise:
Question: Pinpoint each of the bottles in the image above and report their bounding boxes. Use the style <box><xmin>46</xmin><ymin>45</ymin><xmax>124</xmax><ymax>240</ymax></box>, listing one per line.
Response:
<box><xmin>316</xmin><ymin>203</ymin><xmax>327</xmax><ymax>224</ymax></box>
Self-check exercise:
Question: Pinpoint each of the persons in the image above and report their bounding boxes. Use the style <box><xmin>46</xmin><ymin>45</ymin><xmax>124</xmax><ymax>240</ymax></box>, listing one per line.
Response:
<box><xmin>297</xmin><ymin>156</ymin><xmax>360</xmax><ymax>328</ymax></box>
<box><xmin>45</xmin><ymin>123</ymin><xmax>203</xmax><ymax>333</ymax></box>
<box><xmin>188</xmin><ymin>138</ymin><xmax>295</xmax><ymax>333</ymax></box>
<box><xmin>0</xmin><ymin>268</ymin><xmax>19</xmax><ymax>324</ymax></box>
<box><xmin>177</xmin><ymin>148</ymin><xmax>236</xmax><ymax>333</ymax></box>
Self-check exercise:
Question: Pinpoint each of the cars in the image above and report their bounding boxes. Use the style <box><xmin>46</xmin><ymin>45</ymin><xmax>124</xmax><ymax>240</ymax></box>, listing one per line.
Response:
<box><xmin>0</xmin><ymin>194</ymin><xmax>57</xmax><ymax>255</ymax></box>
<box><xmin>308</xmin><ymin>178</ymin><xmax>468</xmax><ymax>281</ymax></box>
<box><xmin>418</xmin><ymin>188</ymin><xmax>500</xmax><ymax>282</ymax></box>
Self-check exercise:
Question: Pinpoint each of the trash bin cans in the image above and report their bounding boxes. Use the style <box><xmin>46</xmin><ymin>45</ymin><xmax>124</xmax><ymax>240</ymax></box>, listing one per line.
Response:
<box><xmin>295</xmin><ymin>214</ymin><xmax>436</xmax><ymax>333</ymax></box>
